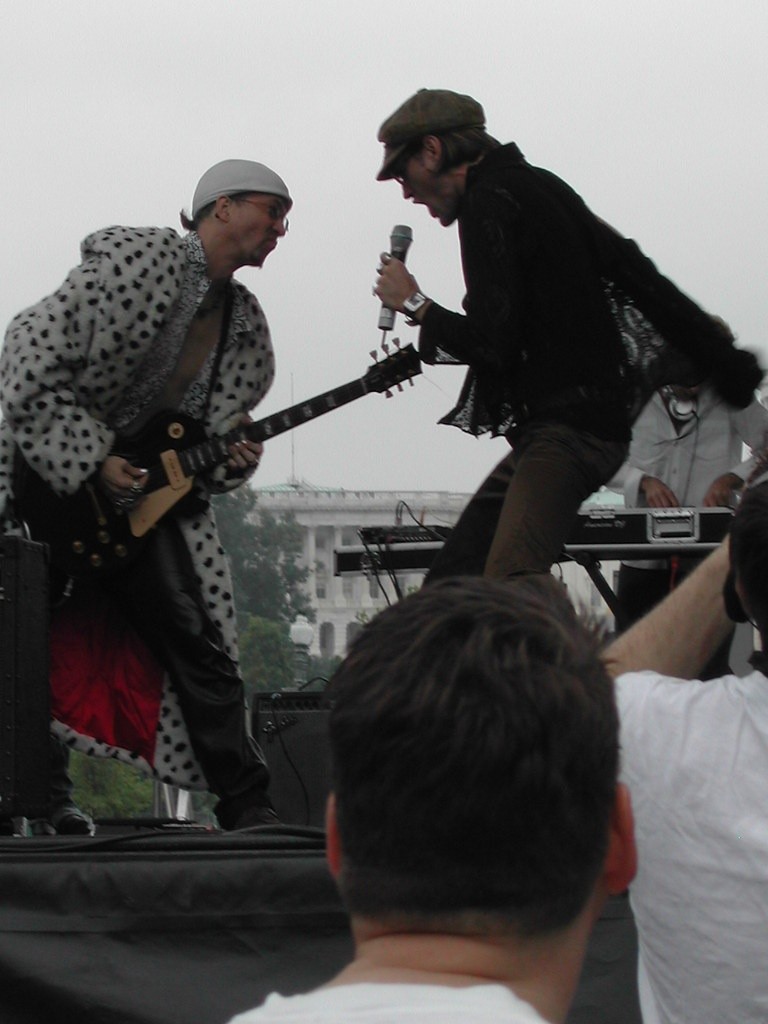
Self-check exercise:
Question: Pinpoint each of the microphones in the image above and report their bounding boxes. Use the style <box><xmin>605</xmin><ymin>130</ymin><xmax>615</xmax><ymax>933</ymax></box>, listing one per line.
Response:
<box><xmin>378</xmin><ymin>226</ymin><xmax>413</xmax><ymax>330</ymax></box>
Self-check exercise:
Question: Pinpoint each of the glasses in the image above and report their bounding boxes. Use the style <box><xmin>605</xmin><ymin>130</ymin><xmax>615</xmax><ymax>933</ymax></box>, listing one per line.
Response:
<box><xmin>391</xmin><ymin>151</ymin><xmax>416</xmax><ymax>184</ymax></box>
<box><xmin>235</xmin><ymin>194</ymin><xmax>288</xmax><ymax>233</ymax></box>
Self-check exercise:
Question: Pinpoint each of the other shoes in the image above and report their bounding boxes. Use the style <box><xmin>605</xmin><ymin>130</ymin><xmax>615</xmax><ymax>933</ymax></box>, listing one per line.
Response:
<box><xmin>235</xmin><ymin>807</ymin><xmax>282</xmax><ymax>831</ymax></box>
<box><xmin>40</xmin><ymin>813</ymin><xmax>95</xmax><ymax>836</ymax></box>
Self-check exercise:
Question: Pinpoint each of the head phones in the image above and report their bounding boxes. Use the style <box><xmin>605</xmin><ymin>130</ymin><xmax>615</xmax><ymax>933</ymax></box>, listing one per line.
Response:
<box><xmin>662</xmin><ymin>384</ymin><xmax>699</xmax><ymax>421</ymax></box>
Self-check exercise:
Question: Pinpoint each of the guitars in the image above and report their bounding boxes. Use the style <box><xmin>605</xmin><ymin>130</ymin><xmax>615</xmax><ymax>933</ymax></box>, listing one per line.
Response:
<box><xmin>13</xmin><ymin>338</ymin><xmax>425</xmax><ymax>585</ymax></box>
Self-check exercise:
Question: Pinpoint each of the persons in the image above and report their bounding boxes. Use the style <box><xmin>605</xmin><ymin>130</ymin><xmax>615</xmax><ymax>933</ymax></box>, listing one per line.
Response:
<box><xmin>1</xmin><ymin>159</ymin><xmax>291</xmax><ymax>835</ymax></box>
<box><xmin>605</xmin><ymin>385</ymin><xmax>768</xmax><ymax>510</ymax></box>
<box><xmin>218</xmin><ymin>575</ymin><xmax>638</xmax><ymax>1023</ymax></box>
<box><xmin>604</xmin><ymin>455</ymin><xmax>768</xmax><ymax>1024</ymax></box>
<box><xmin>372</xmin><ymin>86</ymin><xmax>768</xmax><ymax>681</ymax></box>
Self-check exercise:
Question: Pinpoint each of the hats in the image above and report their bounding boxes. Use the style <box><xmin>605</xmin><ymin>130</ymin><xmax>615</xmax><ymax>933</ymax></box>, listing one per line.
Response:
<box><xmin>192</xmin><ymin>159</ymin><xmax>293</xmax><ymax>216</ymax></box>
<box><xmin>375</xmin><ymin>91</ymin><xmax>486</xmax><ymax>182</ymax></box>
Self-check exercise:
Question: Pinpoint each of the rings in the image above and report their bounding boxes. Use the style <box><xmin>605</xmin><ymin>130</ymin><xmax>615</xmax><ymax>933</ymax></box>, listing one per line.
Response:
<box><xmin>251</xmin><ymin>460</ymin><xmax>259</xmax><ymax>468</ymax></box>
<box><xmin>114</xmin><ymin>499</ymin><xmax>121</xmax><ymax>508</ymax></box>
<box><xmin>383</xmin><ymin>254</ymin><xmax>391</xmax><ymax>264</ymax></box>
<box><xmin>377</xmin><ymin>265</ymin><xmax>383</xmax><ymax>273</ymax></box>
<box><xmin>120</xmin><ymin>498</ymin><xmax>135</xmax><ymax>504</ymax></box>
<box><xmin>131</xmin><ymin>483</ymin><xmax>143</xmax><ymax>495</ymax></box>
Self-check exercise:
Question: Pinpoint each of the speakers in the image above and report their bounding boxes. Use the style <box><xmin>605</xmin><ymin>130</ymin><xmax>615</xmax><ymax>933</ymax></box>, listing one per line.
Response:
<box><xmin>0</xmin><ymin>534</ymin><xmax>54</xmax><ymax>822</ymax></box>
<box><xmin>252</xmin><ymin>691</ymin><xmax>337</xmax><ymax>829</ymax></box>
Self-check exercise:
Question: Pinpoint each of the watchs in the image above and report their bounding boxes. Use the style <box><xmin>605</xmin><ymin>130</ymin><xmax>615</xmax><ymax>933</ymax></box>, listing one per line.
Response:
<box><xmin>403</xmin><ymin>291</ymin><xmax>433</xmax><ymax>327</ymax></box>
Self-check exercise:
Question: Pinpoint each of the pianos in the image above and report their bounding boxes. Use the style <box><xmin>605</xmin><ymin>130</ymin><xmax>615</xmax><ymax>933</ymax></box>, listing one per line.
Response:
<box><xmin>564</xmin><ymin>506</ymin><xmax>736</xmax><ymax>549</ymax></box>
